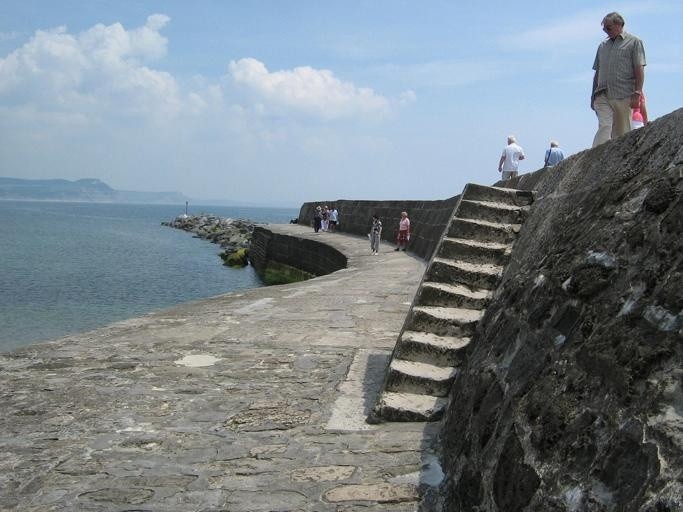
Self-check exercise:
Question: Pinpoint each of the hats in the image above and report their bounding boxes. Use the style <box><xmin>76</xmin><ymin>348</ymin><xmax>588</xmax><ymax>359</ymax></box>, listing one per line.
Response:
<box><xmin>316</xmin><ymin>206</ymin><xmax>321</xmax><ymax>210</ymax></box>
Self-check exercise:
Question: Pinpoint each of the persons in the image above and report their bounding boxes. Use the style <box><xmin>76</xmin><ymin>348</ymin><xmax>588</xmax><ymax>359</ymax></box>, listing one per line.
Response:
<box><xmin>314</xmin><ymin>205</ymin><xmax>339</xmax><ymax>233</ymax></box>
<box><xmin>498</xmin><ymin>134</ymin><xmax>525</xmax><ymax>180</ymax></box>
<box><xmin>544</xmin><ymin>141</ymin><xmax>564</xmax><ymax>166</ymax></box>
<box><xmin>369</xmin><ymin>214</ymin><xmax>382</xmax><ymax>255</ymax></box>
<box><xmin>394</xmin><ymin>211</ymin><xmax>411</xmax><ymax>251</ymax></box>
<box><xmin>589</xmin><ymin>11</ymin><xmax>646</xmax><ymax>148</ymax></box>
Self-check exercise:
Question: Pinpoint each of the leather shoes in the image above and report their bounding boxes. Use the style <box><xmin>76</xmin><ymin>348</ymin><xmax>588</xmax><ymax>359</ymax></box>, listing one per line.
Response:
<box><xmin>394</xmin><ymin>248</ymin><xmax>407</xmax><ymax>251</ymax></box>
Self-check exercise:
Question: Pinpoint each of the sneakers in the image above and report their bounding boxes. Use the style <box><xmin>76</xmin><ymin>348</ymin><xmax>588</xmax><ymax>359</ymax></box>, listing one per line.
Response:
<box><xmin>371</xmin><ymin>249</ymin><xmax>379</xmax><ymax>256</ymax></box>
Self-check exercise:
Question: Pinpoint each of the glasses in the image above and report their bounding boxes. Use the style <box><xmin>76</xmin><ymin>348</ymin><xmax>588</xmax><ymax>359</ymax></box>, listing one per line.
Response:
<box><xmin>603</xmin><ymin>27</ymin><xmax>613</xmax><ymax>31</ymax></box>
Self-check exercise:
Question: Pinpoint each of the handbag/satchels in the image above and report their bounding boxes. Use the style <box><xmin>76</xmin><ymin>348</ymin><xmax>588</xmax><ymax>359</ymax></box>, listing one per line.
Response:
<box><xmin>406</xmin><ymin>232</ymin><xmax>411</xmax><ymax>241</ymax></box>
<box><xmin>544</xmin><ymin>163</ymin><xmax>549</xmax><ymax>167</ymax></box>
